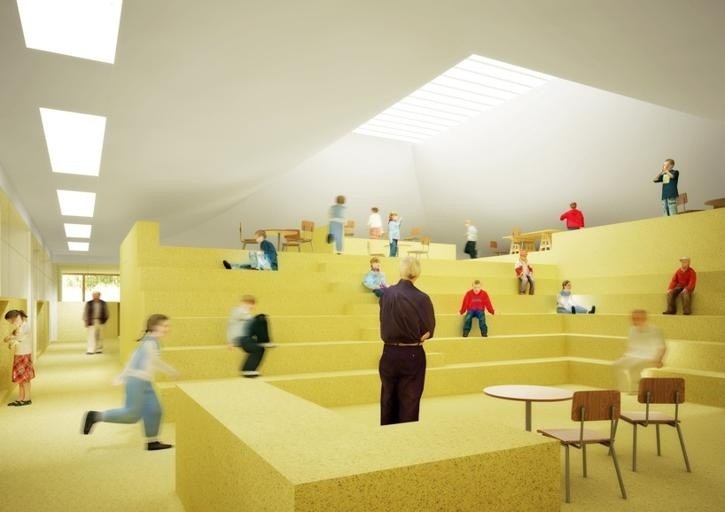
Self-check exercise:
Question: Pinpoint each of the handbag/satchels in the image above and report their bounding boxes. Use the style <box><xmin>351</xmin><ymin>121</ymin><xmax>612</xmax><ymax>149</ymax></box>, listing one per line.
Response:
<box><xmin>327</xmin><ymin>233</ymin><xmax>334</xmax><ymax>244</ymax></box>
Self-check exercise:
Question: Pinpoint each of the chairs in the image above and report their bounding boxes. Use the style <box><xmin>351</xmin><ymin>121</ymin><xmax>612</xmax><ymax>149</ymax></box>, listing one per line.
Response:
<box><xmin>512</xmin><ymin>228</ymin><xmax>534</xmax><ymax>254</ymax></box>
<box><xmin>287</xmin><ymin>221</ymin><xmax>315</xmax><ymax>252</ymax></box>
<box><xmin>619</xmin><ymin>377</ymin><xmax>692</xmax><ymax>473</ymax></box>
<box><xmin>676</xmin><ymin>193</ymin><xmax>704</xmax><ymax>214</ymax></box>
<box><xmin>407</xmin><ymin>236</ymin><xmax>431</xmax><ymax>258</ymax></box>
<box><xmin>240</xmin><ymin>222</ymin><xmax>259</xmax><ymax>250</ymax></box>
<box><xmin>366</xmin><ymin>241</ymin><xmax>383</xmax><ymax>256</ymax></box>
<box><xmin>282</xmin><ymin>229</ymin><xmax>300</xmax><ymax>252</ymax></box>
<box><xmin>404</xmin><ymin>227</ymin><xmax>420</xmax><ymax>241</ymax></box>
<box><xmin>537</xmin><ymin>390</ymin><xmax>627</xmax><ymax>504</ymax></box>
<box><xmin>490</xmin><ymin>241</ymin><xmax>504</xmax><ymax>255</ymax></box>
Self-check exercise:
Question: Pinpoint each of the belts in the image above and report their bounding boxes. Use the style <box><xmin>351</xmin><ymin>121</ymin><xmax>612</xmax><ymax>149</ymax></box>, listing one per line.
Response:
<box><xmin>384</xmin><ymin>341</ymin><xmax>420</xmax><ymax>345</ymax></box>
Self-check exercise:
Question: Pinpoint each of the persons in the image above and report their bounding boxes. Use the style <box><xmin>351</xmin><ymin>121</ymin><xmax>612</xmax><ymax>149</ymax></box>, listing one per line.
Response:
<box><xmin>222</xmin><ymin>230</ymin><xmax>277</xmax><ymax>271</ymax></box>
<box><xmin>613</xmin><ymin>308</ymin><xmax>667</xmax><ymax>395</ymax></box>
<box><xmin>361</xmin><ymin>257</ymin><xmax>388</xmax><ymax>297</ymax></box>
<box><xmin>514</xmin><ymin>250</ymin><xmax>535</xmax><ymax>295</ymax></box>
<box><xmin>83</xmin><ymin>292</ymin><xmax>110</xmax><ymax>354</ymax></box>
<box><xmin>379</xmin><ymin>257</ymin><xmax>435</xmax><ymax>425</ymax></box>
<box><xmin>368</xmin><ymin>207</ymin><xmax>384</xmax><ymax>239</ymax></box>
<box><xmin>459</xmin><ymin>279</ymin><xmax>494</xmax><ymax>337</ymax></box>
<box><xmin>226</xmin><ymin>295</ymin><xmax>277</xmax><ymax>377</ymax></box>
<box><xmin>560</xmin><ymin>202</ymin><xmax>584</xmax><ymax>230</ymax></box>
<box><xmin>662</xmin><ymin>256</ymin><xmax>696</xmax><ymax>315</ymax></box>
<box><xmin>3</xmin><ymin>310</ymin><xmax>35</xmax><ymax>407</ymax></box>
<box><xmin>326</xmin><ymin>196</ymin><xmax>347</xmax><ymax>255</ymax></box>
<box><xmin>556</xmin><ymin>280</ymin><xmax>595</xmax><ymax>315</ymax></box>
<box><xmin>654</xmin><ymin>158</ymin><xmax>679</xmax><ymax>216</ymax></box>
<box><xmin>387</xmin><ymin>212</ymin><xmax>402</xmax><ymax>258</ymax></box>
<box><xmin>465</xmin><ymin>219</ymin><xmax>478</xmax><ymax>258</ymax></box>
<box><xmin>83</xmin><ymin>315</ymin><xmax>177</xmax><ymax>451</ymax></box>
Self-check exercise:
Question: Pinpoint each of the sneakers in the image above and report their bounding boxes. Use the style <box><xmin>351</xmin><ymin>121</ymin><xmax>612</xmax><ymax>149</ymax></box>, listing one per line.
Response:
<box><xmin>148</xmin><ymin>441</ymin><xmax>172</xmax><ymax>450</ymax></box>
<box><xmin>223</xmin><ymin>261</ymin><xmax>231</xmax><ymax>269</ymax></box>
<box><xmin>240</xmin><ymin>370</ymin><xmax>260</xmax><ymax>377</ymax></box>
<box><xmin>8</xmin><ymin>400</ymin><xmax>32</xmax><ymax>406</ymax></box>
<box><xmin>84</xmin><ymin>410</ymin><xmax>96</xmax><ymax>434</ymax></box>
